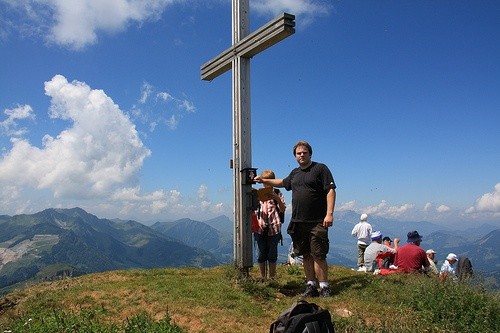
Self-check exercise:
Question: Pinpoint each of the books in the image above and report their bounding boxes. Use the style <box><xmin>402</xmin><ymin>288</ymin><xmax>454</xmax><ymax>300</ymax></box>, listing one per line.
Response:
<box><xmin>258</xmin><ymin>187</ymin><xmax>273</xmax><ymax>201</ymax></box>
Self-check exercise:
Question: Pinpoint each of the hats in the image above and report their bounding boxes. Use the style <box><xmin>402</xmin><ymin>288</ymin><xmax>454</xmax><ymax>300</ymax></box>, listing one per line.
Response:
<box><xmin>407</xmin><ymin>230</ymin><xmax>422</xmax><ymax>242</ymax></box>
<box><xmin>426</xmin><ymin>249</ymin><xmax>437</xmax><ymax>254</ymax></box>
<box><xmin>383</xmin><ymin>236</ymin><xmax>393</xmax><ymax>242</ymax></box>
<box><xmin>371</xmin><ymin>230</ymin><xmax>382</xmax><ymax>240</ymax></box>
<box><xmin>360</xmin><ymin>214</ymin><xmax>368</xmax><ymax>221</ymax></box>
<box><xmin>446</xmin><ymin>253</ymin><xmax>459</xmax><ymax>262</ymax></box>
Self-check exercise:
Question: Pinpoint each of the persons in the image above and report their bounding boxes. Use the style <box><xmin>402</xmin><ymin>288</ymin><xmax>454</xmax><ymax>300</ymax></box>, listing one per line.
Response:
<box><xmin>393</xmin><ymin>229</ymin><xmax>431</xmax><ymax>274</ymax></box>
<box><xmin>363</xmin><ymin>229</ymin><xmax>400</xmax><ymax>271</ymax></box>
<box><xmin>422</xmin><ymin>248</ymin><xmax>438</xmax><ymax>274</ymax></box>
<box><xmin>351</xmin><ymin>213</ymin><xmax>373</xmax><ymax>272</ymax></box>
<box><xmin>252</xmin><ymin>169</ymin><xmax>285</xmax><ymax>283</ymax></box>
<box><xmin>377</xmin><ymin>236</ymin><xmax>396</xmax><ymax>269</ymax></box>
<box><xmin>252</xmin><ymin>140</ymin><xmax>337</xmax><ymax>298</ymax></box>
<box><xmin>440</xmin><ymin>250</ymin><xmax>457</xmax><ymax>285</ymax></box>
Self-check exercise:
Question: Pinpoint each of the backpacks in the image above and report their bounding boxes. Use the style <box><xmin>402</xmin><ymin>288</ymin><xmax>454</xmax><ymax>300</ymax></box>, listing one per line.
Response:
<box><xmin>269</xmin><ymin>300</ymin><xmax>336</xmax><ymax>333</ymax></box>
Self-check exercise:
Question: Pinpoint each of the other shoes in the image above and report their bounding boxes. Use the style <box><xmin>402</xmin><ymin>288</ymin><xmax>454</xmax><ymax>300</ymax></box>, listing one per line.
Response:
<box><xmin>319</xmin><ymin>287</ymin><xmax>332</xmax><ymax>298</ymax></box>
<box><xmin>299</xmin><ymin>285</ymin><xmax>320</xmax><ymax>299</ymax></box>
